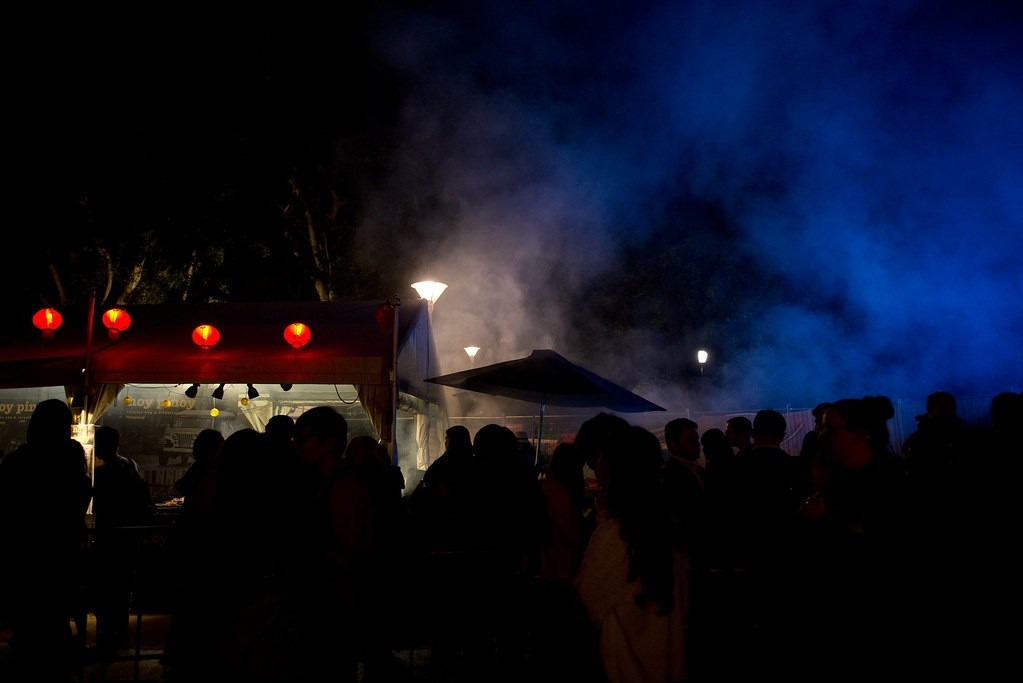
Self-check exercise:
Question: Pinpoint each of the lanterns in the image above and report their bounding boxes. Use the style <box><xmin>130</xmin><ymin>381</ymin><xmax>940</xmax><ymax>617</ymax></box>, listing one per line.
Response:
<box><xmin>103</xmin><ymin>305</ymin><xmax>131</xmax><ymax>339</ymax></box>
<box><xmin>283</xmin><ymin>323</ymin><xmax>311</xmax><ymax>349</ymax></box>
<box><xmin>32</xmin><ymin>308</ymin><xmax>62</xmax><ymax>338</ymax></box>
<box><xmin>191</xmin><ymin>323</ymin><xmax>221</xmax><ymax>350</ymax></box>
<box><xmin>375</xmin><ymin>303</ymin><xmax>401</xmax><ymax>326</ymax></box>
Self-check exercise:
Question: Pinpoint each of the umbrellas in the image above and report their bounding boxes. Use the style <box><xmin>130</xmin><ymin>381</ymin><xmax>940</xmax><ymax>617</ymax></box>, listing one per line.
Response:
<box><xmin>424</xmin><ymin>347</ymin><xmax>666</xmax><ymax>466</ymax></box>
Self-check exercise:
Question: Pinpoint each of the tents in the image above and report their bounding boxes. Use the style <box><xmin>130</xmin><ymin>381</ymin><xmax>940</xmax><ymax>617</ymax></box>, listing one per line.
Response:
<box><xmin>91</xmin><ymin>299</ymin><xmax>447</xmax><ymax>469</ymax></box>
<box><xmin>0</xmin><ymin>302</ymin><xmax>196</xmax><ymax>465</ymax></box>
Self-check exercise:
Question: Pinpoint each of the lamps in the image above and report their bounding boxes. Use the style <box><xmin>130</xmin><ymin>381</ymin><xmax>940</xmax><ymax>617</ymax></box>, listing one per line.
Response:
<box><xmin>34</xmin><ymin>307</ymin><xmax>62</xmax><ymax>339</ymax></box>
<box><xmin>190</xmin><ymin>324</ymin><xmax>220</xmax><ymax>351</ymax></box>
<box><xmin>283</xmin><ymin>321</ymin><xmax>312</xmax><ymax>353</ymax></box>
<box><xmin>101</xmin><ymin>307</ymin><xmax>131</xmax><ymax>341</ymax></box>
<box><xmin>184</xmin><ymin>382</ymin><xmax>293</xmax><ymax>399</ymax></box>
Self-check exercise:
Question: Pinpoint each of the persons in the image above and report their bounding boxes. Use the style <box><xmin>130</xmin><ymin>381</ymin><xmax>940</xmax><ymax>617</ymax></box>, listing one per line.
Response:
<box><xmin>176</xmin><ymin>407</ymin><xmax>544</xmax><ymax>680</ymax></box>
<box><xmin>724</xmin><ymin>418</ymin><xmax>750</xmax><ymax>460</ymax></box>
<box><xmin>804</xmin><ymin>393</ymin><xmax>1023</xmax><ymax>682</ymax></box>
<box><xmin>0</xmin><ymin>398</ymin><xmax>89</xmax><ymax>683</ymax></box>
<box><xmin>699</xmin><ymin>429</ymin><xmax>732</xmax><ymax>481</ymax></box>
<box><xmin>660</xmin><ymin>419</ymin><xmax>705</xmax><ymax>544</ymax></box>
<box><xmin>729</xmin><ymin>405</ymin><xmax>796</xmax><ymax>514</ymax></box>
<box><xmin>89</xmin><ymin>424</ymin><xmax>152</xmax><ymax>660</ymax></box>
<box><xmin>577</xmin><ymin>417</ymin><xmax>650</xmax><ymax>528</ymax></box>
<box><xmin>545</xmin><ymin>446</ymin><xmax>596</xmax><ymax>595</ymax></box>
<box><xmin>567</xmin><ymin>425</ymin><xmax>691</xmax><ymax>683</ymax></box>
